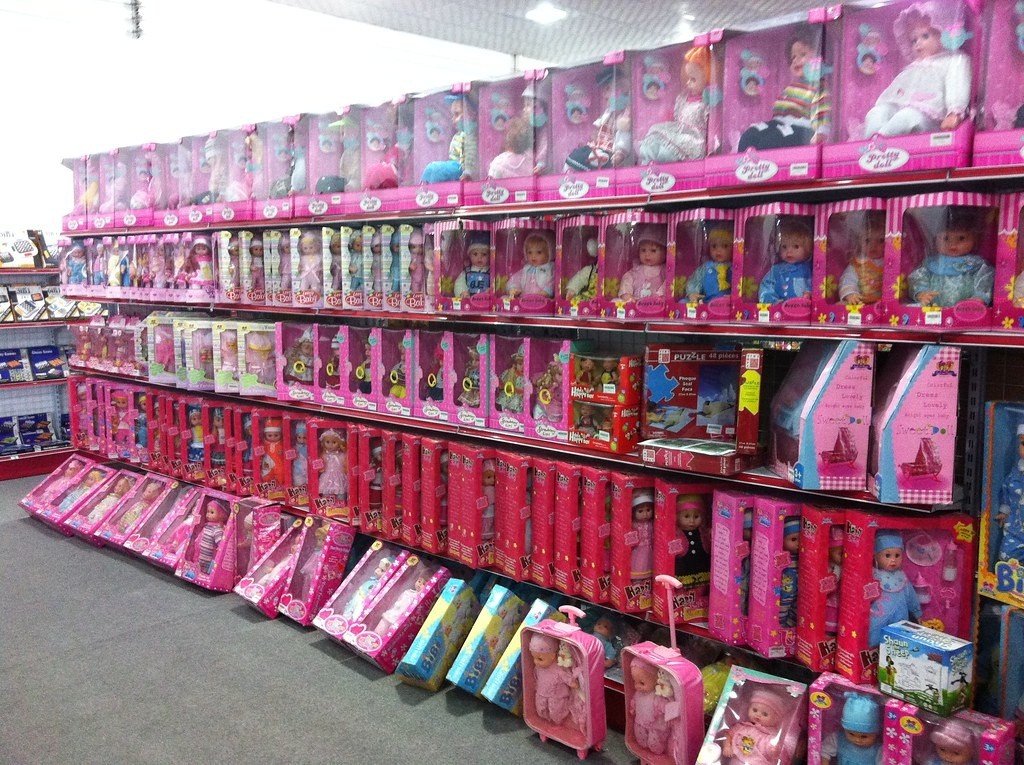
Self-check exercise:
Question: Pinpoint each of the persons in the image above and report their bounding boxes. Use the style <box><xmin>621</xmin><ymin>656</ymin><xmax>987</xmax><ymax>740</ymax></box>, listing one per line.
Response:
<box><xmin>38</xmin><ymin>0</ymin><xmax>1024</xmax><ymax>765</ymax></box>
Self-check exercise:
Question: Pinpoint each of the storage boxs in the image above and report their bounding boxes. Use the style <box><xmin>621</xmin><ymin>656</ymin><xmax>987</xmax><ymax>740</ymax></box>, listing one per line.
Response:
<box><xmin>61</xmin><ymin>189</ymin><xmax>1024</xmax><ymax>334</ymax></box>
<box><xmin>72</xmin><ymin>373</ymin><xmax>976</xmax><ymax>685</ymax></box>
<box><xmin>0</xmin><ymin>413</ymin><xmax>70</xmax><ymax>456</ymax></box>
<box><xmin>61</xmin><ymin>162</ymin><xmax>1024</xmax><ymax>765</ymax></box>
<box><xmin>69</xmin><ymin>312</ymin><xmax>960</xmax><ymax>506</ymax></box>
<box><xmin>18</xmin><ymin>454</ymin><xmax>1017</xmax><ymax>765</ymax></box>
<box><xmin>0</xmin><ymin>225</ymin><xmax>61</xmax><ymax>269</ymax></box>
<box><xmin>1</xmin><ymin>345</ymin><xmax>85</xmax><ymax>383</ymax></box>
<box><xmin>975</xmin><ymin>402</ymin><xmax>1024</xmax><ymax>721</ymax></box>
<box><xmin>1</xmin><ymin>282</ymin><xmax>108</xmax><ymax>323</ymax></box>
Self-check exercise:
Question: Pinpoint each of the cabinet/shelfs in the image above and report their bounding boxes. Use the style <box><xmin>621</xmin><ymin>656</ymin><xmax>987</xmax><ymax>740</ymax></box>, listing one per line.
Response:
<box><xmin>62</xmin><ymin>2</ymin><xmax>1024</xmax><ymax>233</ymax></box>
<box><xmin>0</xmin><ymin>267</ymin><xmax>114</xmax><ymax>481</ymax></box>
